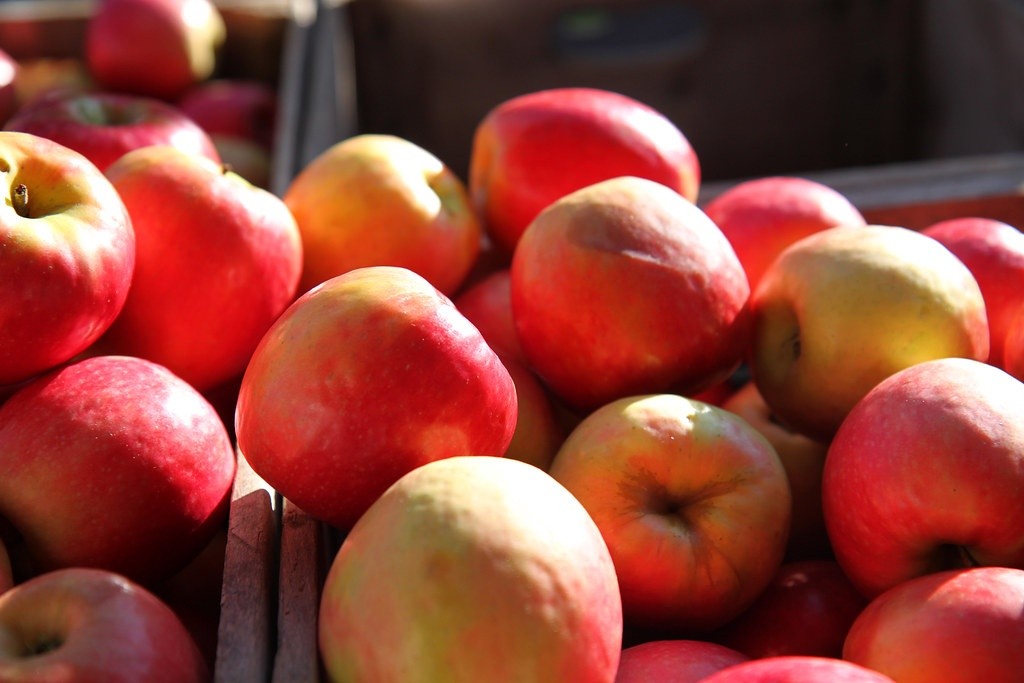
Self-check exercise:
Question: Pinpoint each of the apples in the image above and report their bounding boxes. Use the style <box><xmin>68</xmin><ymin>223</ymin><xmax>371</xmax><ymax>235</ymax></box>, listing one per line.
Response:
<box><xmin>0</xmin><ymin>0</ymin><xmax>1024</xmax><ymax>683</ymax></box>
